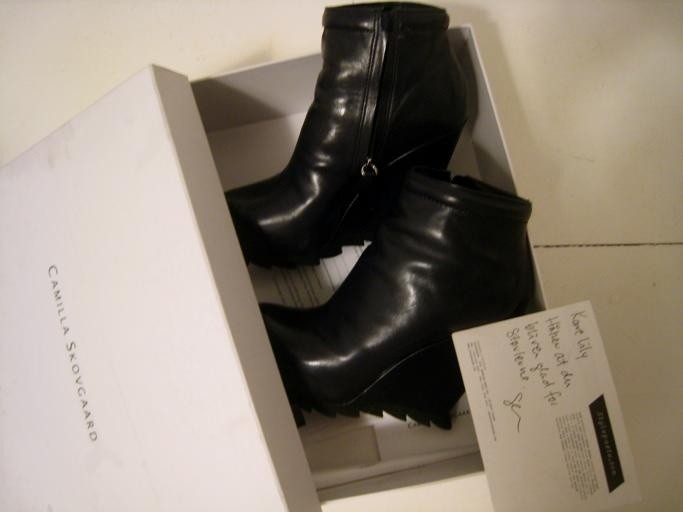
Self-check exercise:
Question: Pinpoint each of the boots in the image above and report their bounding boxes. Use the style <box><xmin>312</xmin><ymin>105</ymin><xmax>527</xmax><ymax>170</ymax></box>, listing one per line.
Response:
<box><xmin>258</xmin><ymin>166</ymin><xmax>532</xmax><ymax>430</ymax></box>
<box><xmin>224</xmin><ymin>3</ymin><xmax>468</xmax><ymax>269</ymax></box>
